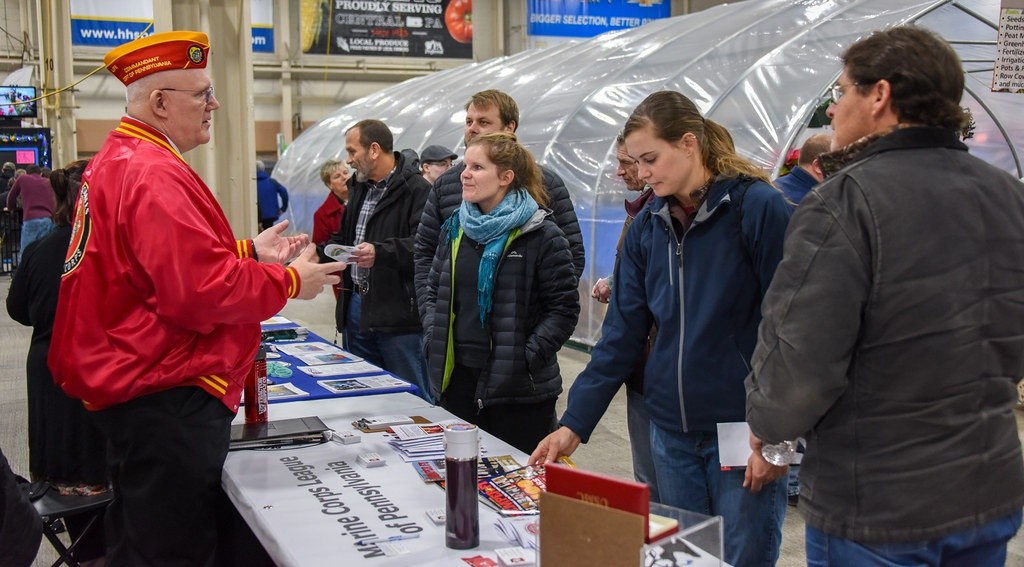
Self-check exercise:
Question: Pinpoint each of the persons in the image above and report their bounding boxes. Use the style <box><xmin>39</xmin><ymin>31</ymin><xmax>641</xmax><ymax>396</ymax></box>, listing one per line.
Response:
<box><xmin>530</xmin><ymin>91</ymin><xmax>794</xmax><ymax>566</ymax></box>
<box><xmin>7</xmin><ymin>160</ymin><xmax>106</xmax><ymax>567</ymax></box>
<box><xmin>310</xmin><ymin>90</ymin><xmax>586</xmax><ymax>456</ymax></box>
<box><xmin>744</xmin><ymin>26</ymin><xmax>1023</xmax><ymax>565</ymax></box>
<box><xmin>47</xmin><ymin>30</ymin><xmax>346</xmax><ymax>567</ymax></box>
<box><xmin>771</xmin><ymin>134</ymin><xmax>837</xmax><ymax>205</ymax></box>
<box><xmin>256</xmin><ymin>160</ymin><xmax>289</xmax><ymax>232</ymax></box>
<box><xmin>0</xmin><ymin>161</ymin><xmax>59</xmax><ymax>266</ymax></box>
<box><xmin>0</xmin><ymin>87</ymin><xmax>36</xmax><ymax>116</ymax></box>
<box><xmin>1</xmin><ymin>449</ymin><xmax>43</xmax><ymax>566</ymax></box>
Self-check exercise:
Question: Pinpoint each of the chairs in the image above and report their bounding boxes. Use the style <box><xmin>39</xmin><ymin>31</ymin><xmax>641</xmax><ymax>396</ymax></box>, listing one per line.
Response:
<box><xmin>0</xmin><ymin>448</ymin><xmax>115</xmax><ymax>567</ymax></box>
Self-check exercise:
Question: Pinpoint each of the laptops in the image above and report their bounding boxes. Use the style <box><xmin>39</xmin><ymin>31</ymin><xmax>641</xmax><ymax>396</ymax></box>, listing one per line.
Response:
<box><xmin>228</xmin><ymin>416</ymin><xmax>330</xmax><ymax>449</ymax></box>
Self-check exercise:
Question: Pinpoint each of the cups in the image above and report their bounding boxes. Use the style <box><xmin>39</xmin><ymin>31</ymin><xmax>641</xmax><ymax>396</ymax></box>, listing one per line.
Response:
<box><xmin>442</xmin><ymin>423</ymin><xmax>480</xmax><ymax>549</ymax></box>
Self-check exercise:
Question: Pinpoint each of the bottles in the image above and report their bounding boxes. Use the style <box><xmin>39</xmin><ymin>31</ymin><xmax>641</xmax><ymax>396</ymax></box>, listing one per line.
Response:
<box><xmin>760</xmin><ymin>440</ymin><xmax>798</xmax><ymax>466</ymax></box>
<box><xmin>244</xmin><ymin>345</ymin><xmax>269</xmax><ymax>424</ymax></box>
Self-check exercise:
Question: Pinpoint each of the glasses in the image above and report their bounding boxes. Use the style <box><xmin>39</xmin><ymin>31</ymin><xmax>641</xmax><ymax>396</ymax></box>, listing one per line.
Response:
<box><xmin>427</xmin><ymin>161</ymin><xmax>456</xmax><ymax>168</ymax></box>
<box><xmin>830</xmin><ymin>80</ymin><xmax>884</xmax><ymax>105</ymax></box>
<box><xmin>160</xmin><ymin>86</ymin><xmax>214</xmax><ymax>103</ymax></box>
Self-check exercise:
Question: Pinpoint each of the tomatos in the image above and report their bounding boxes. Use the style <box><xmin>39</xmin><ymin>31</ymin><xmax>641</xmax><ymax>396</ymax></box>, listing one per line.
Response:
<box><xmin>445</xmin><ymin>0</ymin><xmax>473</xmax><ymax>44</ymax></box>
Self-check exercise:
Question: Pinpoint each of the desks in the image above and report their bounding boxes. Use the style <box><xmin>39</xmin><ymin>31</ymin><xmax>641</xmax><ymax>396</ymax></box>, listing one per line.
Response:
<box><xmin>239</xmin><ymin>314</ymin><xmax>422</xmax><ymax>406</ymax></box>
<box><xmin>221</xmin><ymin>393</ymin><xmax>732</xmax><ymax>567</ymax></box>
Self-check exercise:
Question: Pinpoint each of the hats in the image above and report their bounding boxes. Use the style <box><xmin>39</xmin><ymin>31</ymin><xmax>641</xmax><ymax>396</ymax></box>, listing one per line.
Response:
<box><xmin>420</xmin><ymin>144</ymin><xmax>458</xmax><ymax>166</ymax></box>
<box><xmin>103</xmin><ymin>30</ymin><xmax>211</xmax><ymax>87</ymax></box>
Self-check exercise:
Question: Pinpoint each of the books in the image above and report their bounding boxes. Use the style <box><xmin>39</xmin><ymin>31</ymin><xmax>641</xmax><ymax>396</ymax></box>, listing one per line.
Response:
<box><xmin>387</xmin><ymin>419</ymin><xmax>490</xmax><ymax>462</ymax></box>
<box><xmin>413</xmin><ymin>453</ymin><xmax>588</xmax><ymax>514</ymax></box>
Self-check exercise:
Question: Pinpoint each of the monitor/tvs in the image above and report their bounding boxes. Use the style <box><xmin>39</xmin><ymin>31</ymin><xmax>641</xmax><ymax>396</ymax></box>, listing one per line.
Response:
<box><xmin>0</xmin><ymin>86</ymin><xmax>37</xmax><ymax>118</ymax></box>
